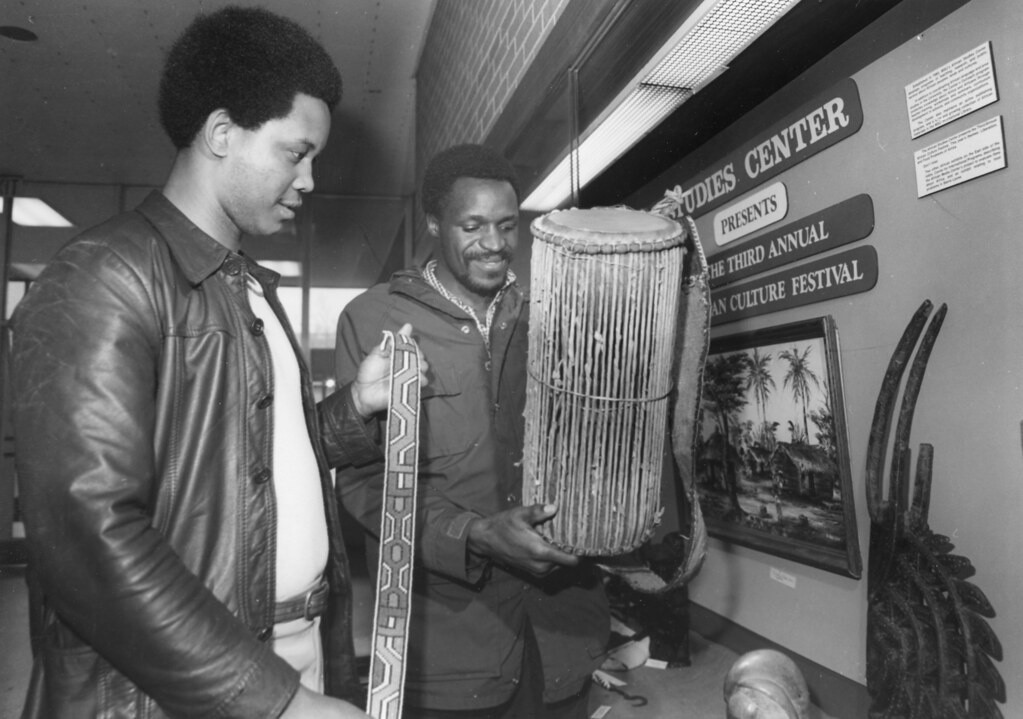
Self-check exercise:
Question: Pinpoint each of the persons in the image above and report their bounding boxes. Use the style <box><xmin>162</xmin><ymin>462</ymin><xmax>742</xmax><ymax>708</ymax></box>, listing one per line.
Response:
<box><xmin>8</xmin><ymin>6</ymin><xmax>373</xmax><ymax>719</ymax></box>
<box><xmin>333</xmin><ymin>144</ymin><xmax>610</xmax><ymax>719</ymax></box>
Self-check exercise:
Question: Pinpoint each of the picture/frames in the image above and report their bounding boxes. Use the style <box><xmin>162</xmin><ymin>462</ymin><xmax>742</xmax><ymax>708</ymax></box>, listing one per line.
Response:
<box><xmin>667</xmin><ymin>314</ymin><xmax>864</xmax><ymax>580</ymax></box>
